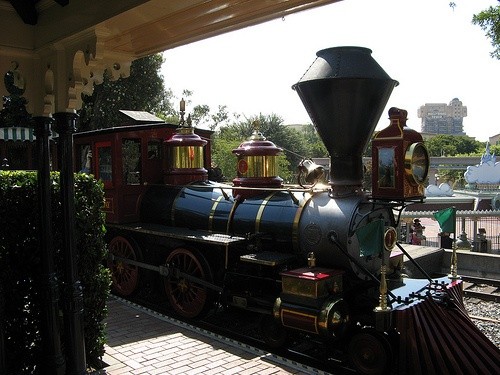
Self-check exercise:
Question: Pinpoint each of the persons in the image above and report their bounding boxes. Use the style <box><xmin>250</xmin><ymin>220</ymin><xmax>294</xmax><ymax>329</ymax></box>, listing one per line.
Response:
<box><xmin>406</xmin><ymin>218</ymin><xmax>425</xmax><ymax>247</ymax></box>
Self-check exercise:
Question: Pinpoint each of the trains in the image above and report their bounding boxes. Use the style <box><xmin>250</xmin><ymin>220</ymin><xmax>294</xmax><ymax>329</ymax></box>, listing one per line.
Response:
<box><xmin>62</xmin><ymin>46</ymin><xmax>499</xmax><ymax>375</ymax></box>
